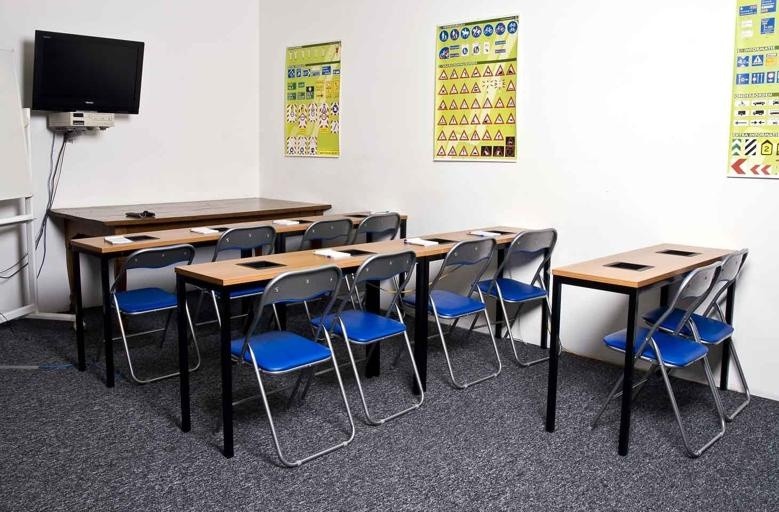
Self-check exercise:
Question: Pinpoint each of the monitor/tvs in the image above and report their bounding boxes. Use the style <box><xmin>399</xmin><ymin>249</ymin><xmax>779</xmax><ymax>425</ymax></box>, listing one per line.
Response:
<box><xmin>32</xmin><ymin>30</ymin><xmax>144</xmax><ymax>114</ymax></box>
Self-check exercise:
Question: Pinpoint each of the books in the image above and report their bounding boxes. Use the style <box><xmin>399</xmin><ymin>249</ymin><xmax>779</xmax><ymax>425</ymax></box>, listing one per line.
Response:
<box><xmin>103</xmin><ymin>234</ymin><xmax>134</xmax><ymax>246</ymax></box>
<box><xmin>313</xmin><ymin>247</ymin><xmax>352</xmax><ymax>261</ymax></box>
<box><xmin>271</xmin><ymin>219</ymin><xmax>300</xmax><ymax>226</ymax></box>
<box><xmin>467</xmin><ymin>229</ymin><xmax>502</xmax><ymax>238</ymax></box>
<box><xmin>190</xmin><ymin>226</ymin><xmax>221</xmax><ymax>235</ymax></box>
<box><xmin>405</xmin><ymin>237</ymin><xmax>441</xmax><ymax>248</ymax></box>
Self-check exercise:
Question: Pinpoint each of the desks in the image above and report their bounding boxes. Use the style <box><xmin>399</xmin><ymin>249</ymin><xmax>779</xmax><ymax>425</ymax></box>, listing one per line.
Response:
<box><xmin>175</xmin><ymin>241</ymin><xmax>426</xmax><ymax>458</ymax></box>
<box><xmin>545</xmin><ymin>243</ymin><xmax>736</xmax><ymax>456</ymax></box>
<box><xmin>42</xmin><ymin>197</ymin><xmax>333</xmax><ymax>331</ymax></box>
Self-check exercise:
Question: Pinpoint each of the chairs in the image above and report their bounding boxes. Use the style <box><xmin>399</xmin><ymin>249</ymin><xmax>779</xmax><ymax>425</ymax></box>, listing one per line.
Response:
<box><xmin>68</xmin><ymin>205</ymin><xmax>562</xmax><ymax>388</ymax></box>
<box><xmin>307</xmin><ymin>250</ymin><xmax>425</xmax><ymax>425</ymax></box>
<box><xmin>639</xmin><ymin>248</ymin><xmax>750</xmax><ymax>421</ymax></box>
<box><xmin>589</xmin><ymin>259</ymin><xmax>727</xmax><ymax>456</ymax></box>
<box><xmin>226</xmin><ymin>264</ymin><xmax>355</xmax><ymax>469</ymax></box>
